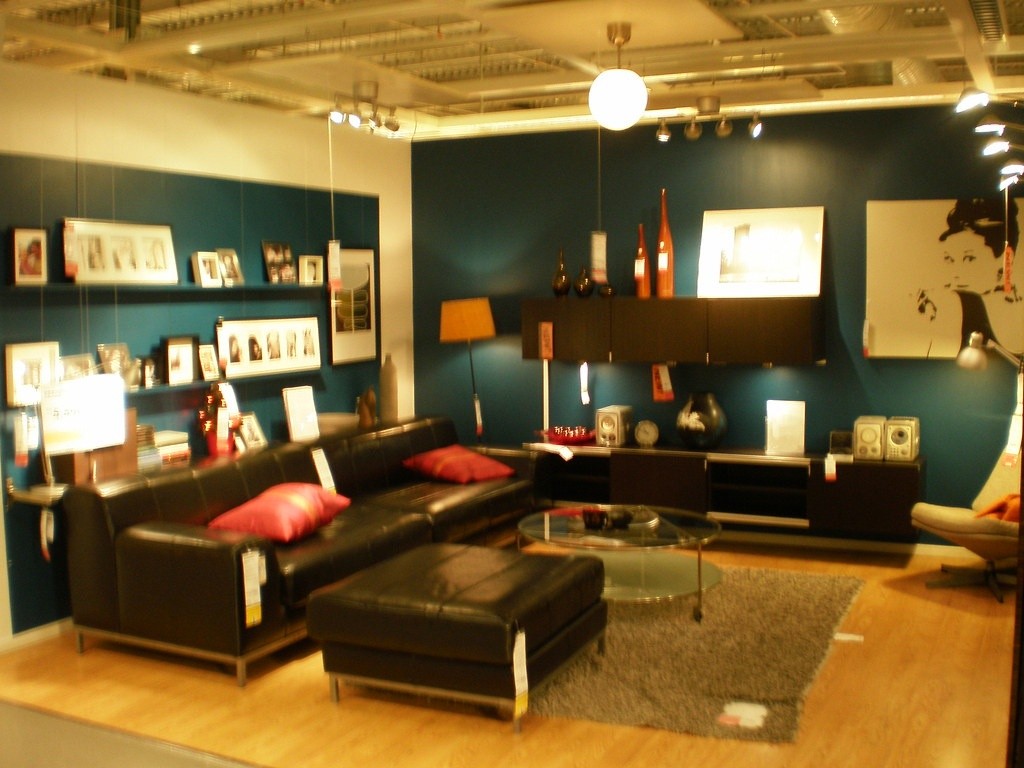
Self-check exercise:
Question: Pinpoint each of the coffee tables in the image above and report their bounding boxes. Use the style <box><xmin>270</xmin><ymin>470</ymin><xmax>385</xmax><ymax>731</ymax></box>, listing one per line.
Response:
<box><xmin>515</xmin><ymin>503</ymin><xmax>721</xmax><ymax>654</ymax></box>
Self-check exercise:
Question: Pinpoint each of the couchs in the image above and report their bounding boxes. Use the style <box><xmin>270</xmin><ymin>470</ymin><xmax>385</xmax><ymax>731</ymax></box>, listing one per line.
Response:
<box><xmin>65</xmin><ymin>417</ymin><xmax>536</xmax><ymax>688</ymax></box>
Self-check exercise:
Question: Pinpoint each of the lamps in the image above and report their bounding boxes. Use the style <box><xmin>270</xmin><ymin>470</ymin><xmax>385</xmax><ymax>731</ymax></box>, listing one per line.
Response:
<box><xmin>327</xmin><ymin>80</ymin><xmax>400</xmax><ymax>132</ymax></box>
<box><xmin>685</xmin><ymin>116</ymin><xmax>704</xmax><ymax>141</ymax></box>
<box><xmin>956</xmin><ymin>332</ymin><xmax>1024</xmax><ymax>374</ymax></box>
<box><xmin>974</xmin><ymin>115</ymin><xmax>1024</xmax><ymax>136</ymax></box>
<box><xmin>439</xmin><ymin>297</ymin><xmax>496</xmax><ymax>446</ymax></box>
<box><xmin>982</xmin><ymin>135</ymin><xmax>1024</xmax><ymax>157</ymax></box>
<box><xmin>588</xmin><ymin>19</ymin><xmax>647</xmax><ymax>132</ymax></box>
<box><xmin>999</xmin><ymin>174</ymin><xmax>1024</xmax><ymax>191</ymax></box>
<box><xmin>1000</xmin><ymin>157</ymin><xmax>1024</xmax><ymax>176</ymax></box>
<box><xmin>953</xmin><ymin>78</ymin><xmax>1024</xmax><ymax>112</ymax></box>
<box><xmin>716</xmin><ymin>115</ymin><xmax>732</xmax><ymax>138</ymax></box>
<box><xmin>656</xmin><ymin>117</ymin><xmax>672</xmax><ymax>143</ymax></box>
<box><xmin>748</xmin><ymin>112</ymin><xmax>763</xmax><ymax>140</ymax></box>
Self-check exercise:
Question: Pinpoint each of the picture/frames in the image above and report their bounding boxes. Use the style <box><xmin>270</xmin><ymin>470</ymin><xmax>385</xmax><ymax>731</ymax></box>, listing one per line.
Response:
<box><xmin>64</xmin><ymin>216</ymin><xmax>182</xmax><ymax>286</ymax></box>
<box><xmin>282</xmin><ymin>386</ymin><xmax>320</xmax><ymax>442</ymax></box>
<box><xmin>239</xmin><ymin>411</ymin><xmax>269</xmax><ymax>450</ymax></box>
<box><xmin>214</xmin><ymin>314</ymin><xmax>323</xmax><ymax>380</ymax></box>
<box><xmin>96</xmin><ymin>342</ymin><xmax>132</xmax><ymax>373</ymax></box>
<box><xmin>190</xmin><ymin>250</ymin><xmax>222</xmax><ymax>288</ymax></box>
<box><xmin>5</xmin><ymin>341</ymin><xmax>59</xmax><ymax>407</ymax></box>
<box><xmin>696</xmin><ymin>207</ymin><xmax>826</xmax><ymax>298</ymax></box>
<box><xmin>59</xmin><ymin>353</ymin><xmax>98</xmax><ymax>381</ymax></box>
<box><xmin>215</xmin><ymin>248</ymin><xmax>245</xmax><ymax>288</ymax></box>
<box><xmin>324</xmin><ymin>247</ymin><xmax>381</xmax><ymax>368</ymax></box>
<box><xmin>197</xmin><ymin>343</ymin><xmax>222</xmax><ymax>383</ymax></box>
<box><xmin>298</xmin><ymin>255</ymin><xmax>324</xmax><ymax>286</ymax></box>
<box><xmin>10</xmin><ymin>224</ymin><xmax>52</xmax><ymax>286</ymax></box>
<box><xmin>159</xmin><ymin>333</ymin><xmax>199</xmax><ymax>384</ymax></box>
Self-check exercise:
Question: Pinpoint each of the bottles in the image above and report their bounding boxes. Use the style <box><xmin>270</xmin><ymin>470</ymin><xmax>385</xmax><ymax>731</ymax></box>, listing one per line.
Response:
<box><xmin>656</xmin><ymin>189</ymin><xmax>674</xmax><ymax>300</ymax></box>
<box><xmin>574</xmin><ymin>267</ymin><xmax>594</xmax><ymax>296</ymax></box>
<box><xmin>677</xmin><ymin>392</ymin><xmax>726</xmax><ymax>452</ymax></box>
<box><xmin>634</xmin><ymin>224</ymin><xmax>651</xmax><ymax>300</ymax></box>
<box><xmin>552</xmin><ymin>249</ymin><xmax>570</xmax><ymax>296</ymax></box>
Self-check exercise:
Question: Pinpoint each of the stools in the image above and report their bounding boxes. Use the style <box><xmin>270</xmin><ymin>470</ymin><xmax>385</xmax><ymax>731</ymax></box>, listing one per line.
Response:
<box><xmin>306</xmin><ymin>542</ymin><xmax>610</xmax><ymax>733</ymax></box>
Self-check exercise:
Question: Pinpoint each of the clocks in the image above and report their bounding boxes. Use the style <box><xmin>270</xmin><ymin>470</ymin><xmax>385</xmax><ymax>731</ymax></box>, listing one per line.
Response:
<box><xmin>632</xmin><ymin>418</ymin><xmax>663</xmax><ymax>448</ymax></box>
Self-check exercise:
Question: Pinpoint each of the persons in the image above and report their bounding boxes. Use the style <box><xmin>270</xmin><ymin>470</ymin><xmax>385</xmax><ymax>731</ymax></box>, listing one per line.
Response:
<box><xmin>223</xmin><ymin>254</ymin><xmax>238</xmax><ymax>279</ymax></box>
<box><xmin>248</xmin><ymin>338</ymin><xmax>262</xmax><ymax>361</ymax></box>
<box><xmin>600</xmin><ymin>417</ymin><xmax>617</xmax><ymax>441</ymax></box>
<box><xmin>245</xmin><ymin>422</ymin><xmax>260</xmax><ymax>442</ymax></box>
<box><xmin>902</xmin><ymin>195</ymin><xmax>1024</xmax><ymax>358</ymax></box>
<box><xmin>227</xmin><ymin>335</ymin><xmax>242</xmax><ymax>364</ymax></box>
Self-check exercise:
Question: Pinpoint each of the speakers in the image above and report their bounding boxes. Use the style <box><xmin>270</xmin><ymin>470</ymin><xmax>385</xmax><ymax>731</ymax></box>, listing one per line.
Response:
<box><xmin>596</xmin><ymin>405</ymin><xmax>634</xmax><ymax>448</ymax></box>
<box><xmin>884</xmin><ymin>416</ymin><xmax>920</xmax><ymax>462</ymax></box>
<box><xmin>854</xmin><ymin>415</ymin><xmax>886</xmax><ymax>461</ymax></box>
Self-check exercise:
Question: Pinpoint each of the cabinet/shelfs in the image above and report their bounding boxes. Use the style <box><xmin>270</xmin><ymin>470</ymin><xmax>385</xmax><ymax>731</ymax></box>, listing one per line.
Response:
<box><xmin>0</xmin><ymin>282</ymin><xmax>328</xmax><ymax>414</ymax></box>
<box><xmin>474</xmin><ymin>439</ymin><xmax>921</xmax><ymax>557</ymax></box>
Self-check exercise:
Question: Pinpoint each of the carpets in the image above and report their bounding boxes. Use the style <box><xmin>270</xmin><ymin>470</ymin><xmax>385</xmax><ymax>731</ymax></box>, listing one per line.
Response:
<box><xmin>529</xmin><ymin>564</ymin><xmax>863</xmax><ymax>744</ymax></box>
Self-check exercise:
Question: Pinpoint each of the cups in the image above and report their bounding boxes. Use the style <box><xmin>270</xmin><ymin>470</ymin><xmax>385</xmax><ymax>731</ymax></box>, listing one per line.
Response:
<box><xmin>599</xmin><ymin>283</ymin><xmax>613</xmax><ymax>297</ymax></box>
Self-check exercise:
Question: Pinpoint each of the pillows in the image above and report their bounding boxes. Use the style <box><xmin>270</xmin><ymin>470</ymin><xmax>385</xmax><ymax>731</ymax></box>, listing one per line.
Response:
<box><xmin>207</xmin><ymin>481</ymin><xmax>352</xmax><ymax>544</ymax></box>
<box><xmin>403</xmin><ymin>444</ymin><xmax>514</xmax><ymax>485</ymax></box>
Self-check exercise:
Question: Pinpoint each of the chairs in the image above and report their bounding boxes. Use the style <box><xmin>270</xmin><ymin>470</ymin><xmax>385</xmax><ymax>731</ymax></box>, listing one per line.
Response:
<box><xmin>910</xmin><ymin>446</ymin><xmax>1021</xmax><ymax>604</ymax></box>
